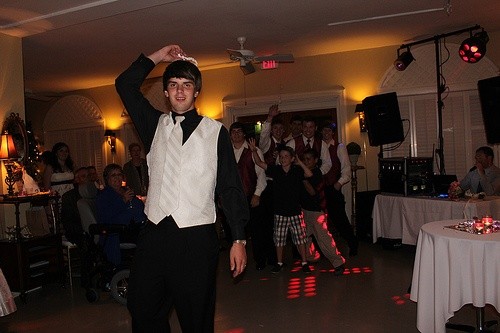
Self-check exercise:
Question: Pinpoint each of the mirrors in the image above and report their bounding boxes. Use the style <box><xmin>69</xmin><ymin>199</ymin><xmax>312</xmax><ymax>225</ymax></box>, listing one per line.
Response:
<box><xmin>0</xmin><ymin>112</ymin><xmax>29</xmax><ymax>165</ymax></box>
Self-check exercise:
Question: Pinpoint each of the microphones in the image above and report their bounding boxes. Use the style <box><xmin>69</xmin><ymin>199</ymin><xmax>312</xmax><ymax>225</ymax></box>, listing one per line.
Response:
<box><xmin>469</xmin><ymin>166</ymin><xmax>477</xmax><ymax>172</ymax></box>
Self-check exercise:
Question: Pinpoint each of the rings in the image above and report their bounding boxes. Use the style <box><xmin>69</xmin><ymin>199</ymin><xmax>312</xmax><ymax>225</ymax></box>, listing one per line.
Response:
<box><xmin>245</xmin><ymin>264</ymin><xmax>247</xmax><ymax>267</ymax></box>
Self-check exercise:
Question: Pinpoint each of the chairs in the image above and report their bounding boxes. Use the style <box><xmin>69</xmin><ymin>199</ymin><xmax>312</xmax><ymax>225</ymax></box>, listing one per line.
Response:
<box><xmin>56</xmin><ymin>195</ymin><xmax>80</xmax><ymax>287</ymax></box>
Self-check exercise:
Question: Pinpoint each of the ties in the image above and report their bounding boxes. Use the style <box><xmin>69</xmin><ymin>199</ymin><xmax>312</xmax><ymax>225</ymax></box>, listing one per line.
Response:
<box><xmin>158</xmin><ymin>116</ymin><xmax>185</xmax><ymax>217</ymax></box>
<box><xmin>477</xmin><ymin>180</ymin><xmax>484</xmax><ymax>193</ymax></box>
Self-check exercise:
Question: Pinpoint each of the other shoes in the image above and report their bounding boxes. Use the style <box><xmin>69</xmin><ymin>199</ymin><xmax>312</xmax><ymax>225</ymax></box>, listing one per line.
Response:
<box><xmin>301</xmin><ymin>264</ymin><xmax>311</xmax><ymax>274</ymax></box>
<box><xmin>350</xmin><ymin>244</ymin><xmax>359</xmax><ymax>256</ymax></box>
<box><xmin>255</xmin><ymin>255</ymin><xmax>265</xmax><ymax>270</ymax></box>
<box><xmin>273</xmin><ymin>263</ymin><xmax>283</xmax><ymax>274</ymax></box>
<box><xmin>335</xmin><ymin>265</ymin><xmax>344</xmax><ymax>275</ymax></box>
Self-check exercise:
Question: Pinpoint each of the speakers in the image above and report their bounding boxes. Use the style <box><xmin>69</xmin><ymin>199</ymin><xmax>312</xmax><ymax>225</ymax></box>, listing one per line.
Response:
<box><xmin>363</xmin><ymin>92</ymin><xmax>405</xmax><ymax>146</ymax></box>
<box><xmin>477</xmin><ymin>77</ymin><xmax>500</xmax><ymax>146</ymax></box>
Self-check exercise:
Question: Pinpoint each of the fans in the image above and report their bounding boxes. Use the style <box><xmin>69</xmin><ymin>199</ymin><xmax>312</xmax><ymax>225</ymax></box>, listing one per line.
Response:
<box><xmin>227</xmin><ymin>37</ymin><xmax>295</xmax><ymax>76</ymax></box>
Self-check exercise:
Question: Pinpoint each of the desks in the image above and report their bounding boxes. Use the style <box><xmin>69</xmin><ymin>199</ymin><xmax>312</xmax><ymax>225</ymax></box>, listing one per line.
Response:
<box><xmin>372</xmin><ymin>194</ymin><xmax>500</xmax><ymax>248</ymax></box>
<box><xmin>409</xmin><ymin>219</ymin><xmax>500</xmax><ymax>333</ymax></box>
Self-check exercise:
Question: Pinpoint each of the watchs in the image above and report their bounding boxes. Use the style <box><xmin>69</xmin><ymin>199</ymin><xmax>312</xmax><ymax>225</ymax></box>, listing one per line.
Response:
<box><xmin>233</xmin><ymin>240</ymin><xmax>246</xmax><ymax>246</ymax></box>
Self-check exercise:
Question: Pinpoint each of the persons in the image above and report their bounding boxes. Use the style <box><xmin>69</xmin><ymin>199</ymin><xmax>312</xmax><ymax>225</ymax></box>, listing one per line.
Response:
<box><xmin>33</xmin><ymin>104</ymin><xmax>354</xmax><ymax>282</ymax></box>
<box><xmin>450</xmin><ymin>146</ymin><xmax>500</xmax><ymax>197</ymax></box>
<box><xmin>113</xmin><ymin>45</ymin><xmax>250</xmax><ymax>333</ymax></box>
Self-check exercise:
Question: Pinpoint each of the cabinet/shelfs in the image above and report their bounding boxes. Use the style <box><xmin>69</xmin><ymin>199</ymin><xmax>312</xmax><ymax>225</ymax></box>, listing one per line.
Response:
<box><xmin>0</xmin><ymin>194</ymin><xmax>62</xmax><ymax>304</ymax></box>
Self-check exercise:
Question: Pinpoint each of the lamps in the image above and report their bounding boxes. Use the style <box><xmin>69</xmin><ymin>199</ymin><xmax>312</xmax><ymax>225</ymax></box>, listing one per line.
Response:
<box><xmin>0</xmin><ymin>130</ymin><xmax>23</xmax><ymax>197</ymax></box>
<box><xmin>104</xmin><ymin>129</ymin><xmax>116</xmax><ymax>151</ymax></box>
<box><xmin>458</xmin><ymin>30</ymin><xmax>490</xmax><ymax>64</ymax></box>
<box><xmin>394</xmin><ymin>46</ymin><xmax>415</xmax><ymax>72</ymax></box>
